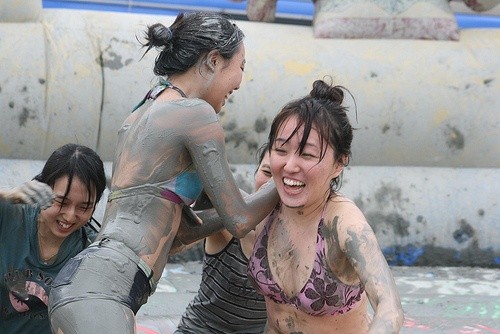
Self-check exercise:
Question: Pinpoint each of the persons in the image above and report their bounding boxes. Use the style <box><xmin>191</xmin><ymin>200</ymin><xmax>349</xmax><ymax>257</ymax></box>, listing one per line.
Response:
<box><xmin>174</xmin><ymin>143</ymin><xmax>273</xmax><ymax>334</ymax></box>
<box><xmin>246</xmin><ymin>79</ymin><xmax>404</xmax><ymax>334</ymax></box>
<box><xmin>0</xmin><ymin>143</ymin><xmax>107</xmax><ymax>334</ymax></box>
<box><xmin>47</xmin><ymin>10</ymin><xmax>280</xmax><ymax>334</ymax></box>
